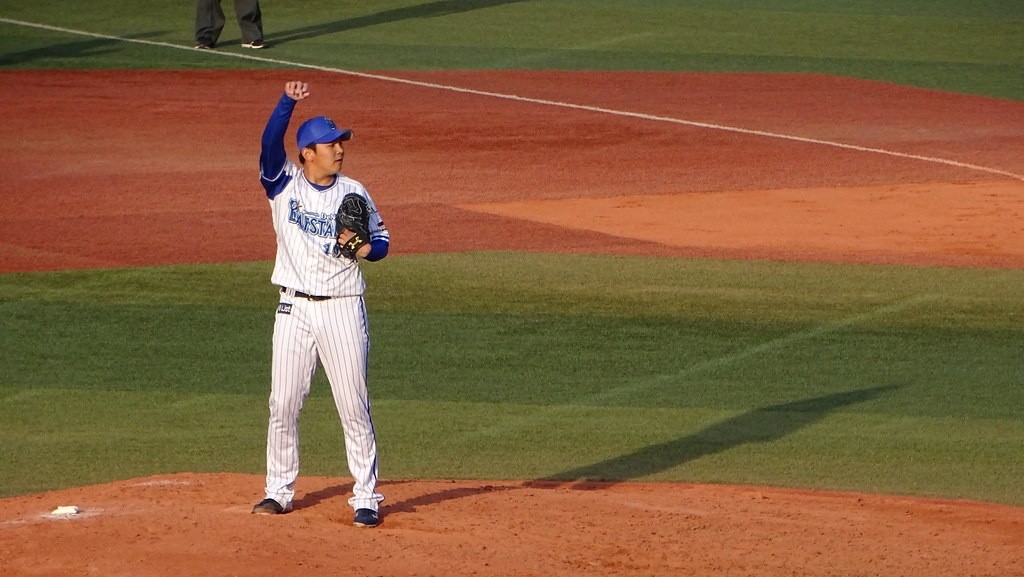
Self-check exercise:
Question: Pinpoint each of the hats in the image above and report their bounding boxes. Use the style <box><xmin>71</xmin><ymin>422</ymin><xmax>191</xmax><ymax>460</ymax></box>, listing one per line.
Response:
<box><xmin>297</xmin><ymin>116</ymin><xmax>353</xmax><ymax>149</ymax></box>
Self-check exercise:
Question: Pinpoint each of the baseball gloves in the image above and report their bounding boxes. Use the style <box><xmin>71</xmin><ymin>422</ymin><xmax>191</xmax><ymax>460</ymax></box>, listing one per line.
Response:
<box><xmin>333</xmin><ymin>193</ymin><xmax>371</xmax><ymax>260</ymax></box>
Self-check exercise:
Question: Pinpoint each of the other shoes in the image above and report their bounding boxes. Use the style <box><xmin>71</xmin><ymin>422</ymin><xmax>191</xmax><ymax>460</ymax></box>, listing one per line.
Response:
<box><xmin>241</xmin><ymin>40</ymin><xmax>265</xmax><ymax>49</ymax></box>
<box><xmin>352</xmin><ymin>509</ymin><xmax>378</xmax><ymax>527</ymax></box>
<box><xmin>252</xmin><ymin>499</ymin><xmax>283</xmax><ymax>514</ymax></box>
<box><xmin>194</xmin><ymin>43</ymin><xmax>210</xmax><ymax>49</ymax></box>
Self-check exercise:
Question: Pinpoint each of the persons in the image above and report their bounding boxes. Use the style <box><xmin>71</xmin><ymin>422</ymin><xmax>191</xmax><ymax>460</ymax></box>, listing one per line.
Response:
<box><xmin>251</xmin><ymin>81</ymin><xmax>389</xmax><ymax>528</ymax></box>
<box><xmin>193</xmin><ymin>0</ymin><xmax>268</xmax><ymax>50</ymax></box>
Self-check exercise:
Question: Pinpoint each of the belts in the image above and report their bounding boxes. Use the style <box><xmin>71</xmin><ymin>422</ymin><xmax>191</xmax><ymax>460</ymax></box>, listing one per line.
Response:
<box><xmin>282</xmin><ymin>286</ymin><xmax>332</xmax><ymax>301</ymax></box>
